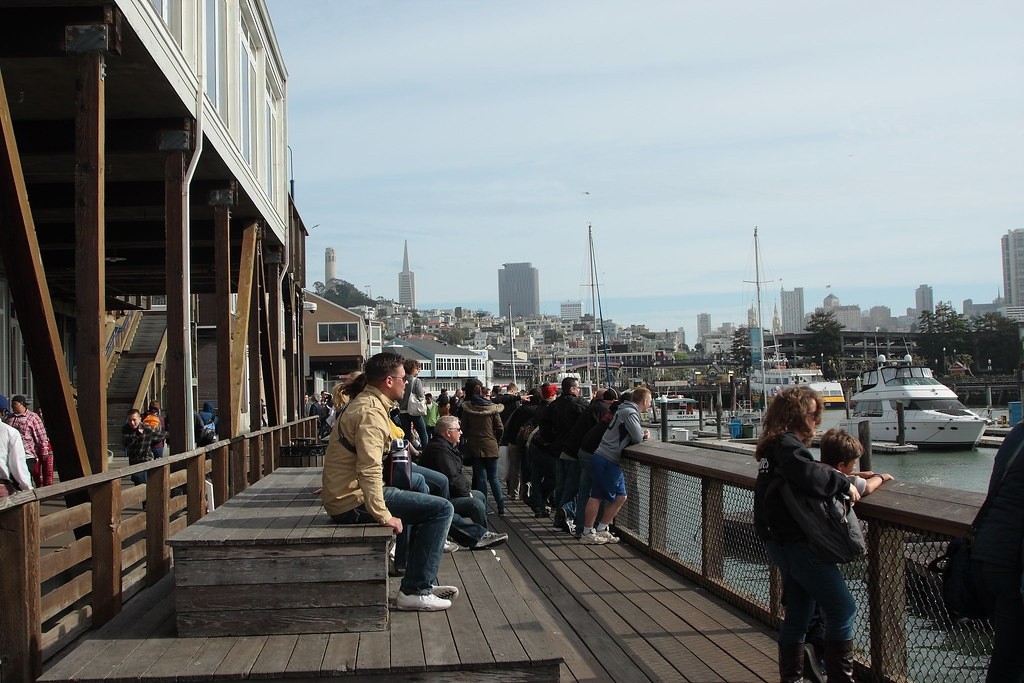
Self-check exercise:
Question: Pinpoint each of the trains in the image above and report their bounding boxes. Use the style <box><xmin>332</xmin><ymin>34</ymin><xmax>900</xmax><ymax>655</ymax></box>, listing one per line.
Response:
<box><xmin>493</xmin><ymin>360</ymin><xmax>533</xmax><ymax>367</ymax></box>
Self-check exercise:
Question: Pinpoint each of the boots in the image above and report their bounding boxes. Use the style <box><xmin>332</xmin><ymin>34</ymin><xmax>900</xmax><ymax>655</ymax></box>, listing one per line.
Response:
<box><xmin>825</xmin><ymin>636</ymin><xmax>855</xmax><ymax>683</ymax></box>
<box><xmin>778</xmin><ymin>640</ymin><xmax>805</xmax><ymax>683</ymax></box>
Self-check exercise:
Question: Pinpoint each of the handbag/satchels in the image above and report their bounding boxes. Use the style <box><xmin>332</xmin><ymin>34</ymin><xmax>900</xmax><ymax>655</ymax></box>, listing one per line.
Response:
<box><xmin>928</xmin><ymin>538</ymin><xmax>989</xmax><ymax>619</ymax></box>
<box><xmin>517</xmin><ymin>420</ymin><xmax>549</xmax><ymax>453</ymax></box>
<box><xmin>408</xmin><ymin>377</ymin><xmax>427</xmax><ymax>416</ymax></box>
<box><xmin>383</xmin><ymin>440</ymin><xmax>412</xmax><ymax>490</ymax></box>
<box><xmin>785</xmin><ymin>495</ymin><xmax>867</xmax><ymax>565</ymax></box>
<box><xmin>462</xmin><ymin>441</ymin><xmax>472</xmax><ymax>466</ymax></box>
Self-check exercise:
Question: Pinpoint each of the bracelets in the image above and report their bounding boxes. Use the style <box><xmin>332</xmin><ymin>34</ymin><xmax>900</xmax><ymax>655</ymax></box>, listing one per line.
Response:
<box><xmin>870</xmin><ymin>474</ymin><xmax>884</xmax><ymax>484</ymax></box>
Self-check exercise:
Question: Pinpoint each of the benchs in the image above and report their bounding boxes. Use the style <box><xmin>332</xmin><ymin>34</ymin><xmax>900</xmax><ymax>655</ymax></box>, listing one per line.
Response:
<box><xmin>164</xmin><ymin>468</ymin><xmax>394</xmax><ymax>640</ymax></box>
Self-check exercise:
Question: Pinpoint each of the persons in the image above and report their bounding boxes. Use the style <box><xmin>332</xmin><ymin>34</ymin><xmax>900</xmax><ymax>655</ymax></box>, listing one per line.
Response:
<box><xmin>983</xmin><ymin>418</ymin><xmax>1024</xmax><ymax>682</ymax></box>
<box><xmin>0</xmin><ymin>393</ymin><xmax>53</xmax><ymax>492</ymax></box>
<box><xmin>320</xmin><ymin>353</ymin><xmax>459</xmax><ymax>611</ymax></box>
<box><xmin>802</xmin><ymin>428</ymin><xmax>894</xmax><ymax>683</ymax></box>
<box><xmin>122</xmin><ymin>400</ymin><xmax>218</xmax><ymax>512</ymax></box>
<box><xmin>753</xmin><ymin>386</ymin><xmax>861</xmax><ymax>683</ymax></box>
<box><xmin>301</xmin><ymin>350</ymin><xmax>653</xmax><ymax>612</ymax></box>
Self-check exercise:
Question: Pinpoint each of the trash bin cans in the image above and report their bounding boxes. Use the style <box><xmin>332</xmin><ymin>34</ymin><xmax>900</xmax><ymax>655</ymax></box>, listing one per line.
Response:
<box><xmin>280</xmin><ymin>438</ymin><xmax>327</xmax><ymax>467</ymax></box>
<box><xmin>741</xmin><ymin>424</ymin><xmax>754</xmax><ymax>438</ymax></box>
<box><xmin>728</xmin><ymin>420</ymin><xmax>741</xmax><ymax>439</ymax></box>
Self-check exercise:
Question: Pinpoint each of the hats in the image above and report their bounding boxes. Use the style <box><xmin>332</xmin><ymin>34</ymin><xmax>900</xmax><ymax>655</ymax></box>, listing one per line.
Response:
<box><xmin>0</xmin><ymin>394</ymin><xmax>7</xmax><ymax>408</ymax></box>
<box><xmin>603</xmin><ymin>388</ymin><xmax>622</xmax><ymax>400</ymax></box>
<box><xmin>12</xmin><ymin>395</ymin><xmax>30</xmax><ymax>405</ymax></box>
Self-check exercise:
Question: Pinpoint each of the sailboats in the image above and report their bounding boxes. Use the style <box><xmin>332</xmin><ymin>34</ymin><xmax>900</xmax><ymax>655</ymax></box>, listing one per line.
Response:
<box><xmin>721</xmin><ymin>224</ymin><xmax>792</xmax><ymax>434</ymax></box>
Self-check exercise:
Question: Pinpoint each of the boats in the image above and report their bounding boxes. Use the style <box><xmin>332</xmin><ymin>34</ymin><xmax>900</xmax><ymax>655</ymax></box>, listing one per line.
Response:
<box><xmin>703</xmin><ymin>399</ymin><xmax>764</xmax><ymax>435</ymax></box>
<box><xmin>837</xmin><ymin>354</ymin><xmax>994</xmax><ymax>451</ymax></box>
<box><xmin>749</xmin><ymin>339</ymin><xmax>845</xmax><ymax>410</ymax></box>
<box><xmin>639</xmin><ymin>395</ymin><xmax>729</xmax><ymax>440</ymax></box>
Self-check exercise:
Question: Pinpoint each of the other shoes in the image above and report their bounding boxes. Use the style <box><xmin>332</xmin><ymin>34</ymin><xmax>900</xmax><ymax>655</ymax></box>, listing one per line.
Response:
<box><xmin>498</xmin><ymin>511</ymin><xmax>506</xmax><ymax>517</ymax></box>
<box><xmin>535</xmin><ymin>496</ymin><xmax>552</xmax><ymax>518</ymax></box>
<box><xmin>486</xmin><ymin>511</ymin><xmax>494</xmax><ymax>515</ymax></box>
<box><xmin>523</xmin><ymin>483</ymin><xmax>531</xmax><ymax>498</ymax></box>
<box><xmin>803</xmin><ymin>643</ymin><xmax>824</xmax><ymax>683</ymax></box>
<box><xmin>510</xmin><ymin>496</ymin><xmax>520</xmax><ymax>500</ymax></box>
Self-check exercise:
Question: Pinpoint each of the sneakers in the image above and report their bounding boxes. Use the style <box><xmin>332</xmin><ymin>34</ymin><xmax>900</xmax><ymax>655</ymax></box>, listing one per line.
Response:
<box><xmin>430</xmin><ymin>585</ymin><xmax>459</xmax><ymax>602</ymax></box>
<box><xmin>474</xmin><ymin>531</ymin><xmax>508</xmax><ymax>548</ymax></box>
<box><xmin>596</xmin><ymin>526</ymin><xmax>620</xmax><ymax>543</ymax></box>
<box><xmin>579</xmin><ymin>528</ymin><xmax>608</xmax><ymax>544</ymax></box>
<box><xmin>396</xmin><ymin>591</ymin><xmax>451</xmax><ymax>611</ymax></box>
<box><xmin>442</xmin><ymin>539</ymin><xmax>459</xmax><ymax>552</ymax></box>
<box><xmin>552</xmin><ymin>506</ymin><xmax>576</xmax><ymax>533</ymax></box>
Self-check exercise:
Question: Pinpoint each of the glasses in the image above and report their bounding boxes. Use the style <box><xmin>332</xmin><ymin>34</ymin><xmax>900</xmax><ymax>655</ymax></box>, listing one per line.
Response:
<box><xmin>448</xmin><ymin>428</ymin><xmax>461</xmax><ymax>432</ymax></box>
<box><xmin>382</xmin><ymin>376</ymin><xmax>407</xmax><ymax>382</ymax></box>
